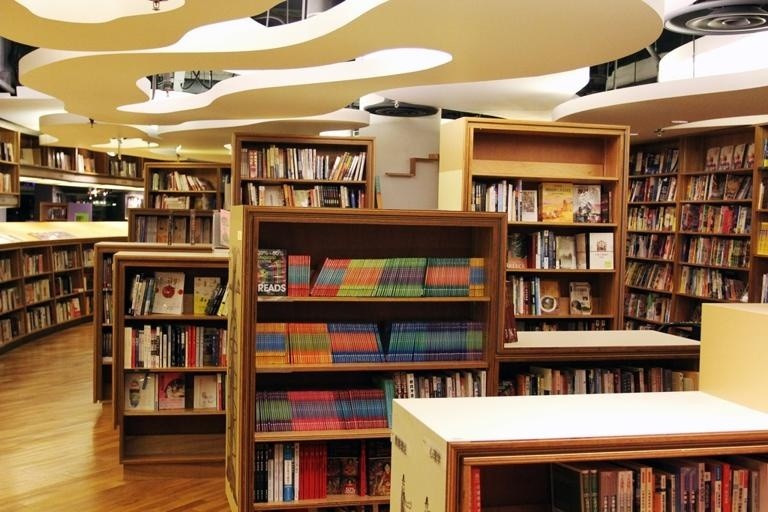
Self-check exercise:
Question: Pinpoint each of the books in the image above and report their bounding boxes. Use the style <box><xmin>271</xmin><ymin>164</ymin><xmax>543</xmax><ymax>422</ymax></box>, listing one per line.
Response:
<box><xmin>0</xmin><ymin>143</ymin><xmax>137</xmax><ymax>221</ymax></box>
<box><xmin>102</xmin><ymin>256</ymin><xmax>228</xmax><ymax>412</ymax></box>
<box><xmin>1</xmin><ymin>249</ymin><xmax>96</xmax><ymax>343</ymax></box>
<box><xmin>240</xmin><ymin>144</ymin><xmax>367</xmax><ymax>209</ymax></box>
<box><xmin>152</xmin><ymin>171</ymin><xmax>232</xmax><ymax>210</ymax></box>
<box><xmin>136</xmin><ymin>216</ymin><xmax>212</xmax><ymax>244</ymax></box>
<box><xmin>499</xmin><ymin>366</ymin><xmax>700</xmax><ymax>396</ymax></box>
<box><xmin>470</xmin><ymin>458</ymin><xmax>767</xmax><ymax>512</ymax></box>
<box><xmin>471</xmin><ymin>178</ymin><xmax>616</xmax><ymax>342</ymax></box>
<box><xmin>254</xmin><ymin>249</ymin><xmax>488</xmax><ymax>504</ymax></box>
<box><xmin>624</xmin><ymin>143</ymin><xmax>768</xmax><ymax>341</ymax></box>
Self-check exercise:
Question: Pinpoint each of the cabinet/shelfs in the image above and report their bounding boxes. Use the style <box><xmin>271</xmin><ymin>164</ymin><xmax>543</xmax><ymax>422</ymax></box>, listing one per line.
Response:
<box><xmin>628</xmin><ymin>122</ymin><xmax>768</xmax><ymax>327</ymax></box>
<box><xmin>438</xmin><ymin>120</ymin><xmax>626</xmax><ymax>327</ymax></box>
<box><xmin>501</xmin><ymin>325</ymin><xmax>700</xmax><ymax>394</ymax></box>
<box><xmin>111</xmin><ymin>253</ymin><xmax>232</xmax><ymax>467</ymax></box>
<box><xmin>91</xmin><ymin>242</ymin><xmax>214</xmax><ymax>403</ymax></box>
<box><xmin>1</xmin><ymin>118</ymin><xmax>20</xmax><ymax>206</ymax></box>
<box><xmin>391</xmin><ymin>393</ymin><xmax>766</xmax><ymax>512</ymax></box>
<box><xmin>221</xmin><ymin>213</ymin><xmax>508</xmax><ymax>506</ymax></box>
<box><xmin>143</xmin><ymin>163</ymin><xmax>230</xmax><ymax>209</ymax></box>
<box><xmin>13</xmin><ymin>132</ymin><xmax>145</xmax><ymax>219</ymax></box>
<box><xmin>229</xmin><ymin>131</ymin><xmax>376</xmax><ymax>210</ymax></box>
<box><xmin>124</xmin><ymin>210</ymin><xmax>217</xmax><ymax>240</ymax></box>
<box><xmin>1</xmin><ymin>219</ymin><xmax>126</xmax><ymax>350</ymax></box>
